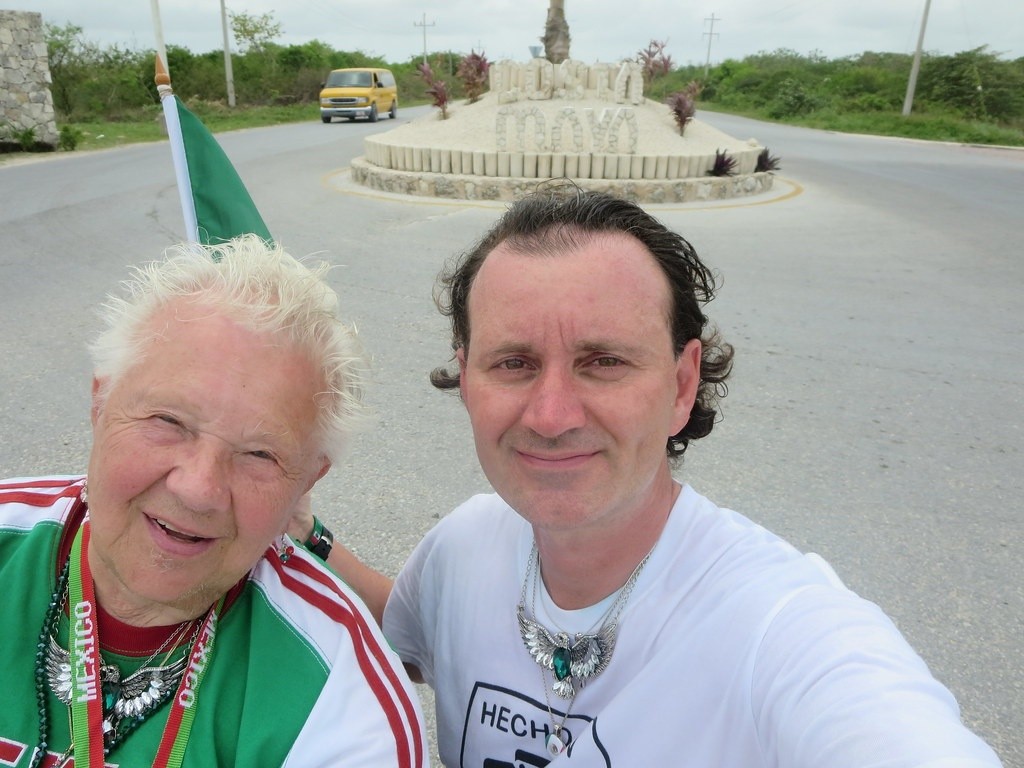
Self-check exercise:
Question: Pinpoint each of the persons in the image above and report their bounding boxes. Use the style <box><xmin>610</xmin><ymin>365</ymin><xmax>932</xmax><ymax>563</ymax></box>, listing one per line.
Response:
<box><xmin>0</xmin><ymin>233</ymin><xmax>431</xmax><ymax>768</ymax></box>
<box><xmin>285</xmin><ymin>192</ymin><xmax>1004</xmax><ymax>768</ymax></box>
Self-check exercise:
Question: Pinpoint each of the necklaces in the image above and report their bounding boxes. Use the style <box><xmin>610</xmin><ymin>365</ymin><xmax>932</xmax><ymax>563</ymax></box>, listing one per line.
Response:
<box><xmin>32</xmin><ymin>554</ymin><xmax>205</xmax><ymax>768</ymax></box>
<box><xmin>515</xmin><ymin>538</ymin><xmax>657</xmax><ymax>763</ymax></box>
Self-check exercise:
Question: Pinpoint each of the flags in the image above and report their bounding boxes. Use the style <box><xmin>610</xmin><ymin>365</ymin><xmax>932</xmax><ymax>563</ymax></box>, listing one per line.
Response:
<box><xmin>173</xmin><ymin>96</ymin><xmax>275</xmax><ymax>259</ymax></box>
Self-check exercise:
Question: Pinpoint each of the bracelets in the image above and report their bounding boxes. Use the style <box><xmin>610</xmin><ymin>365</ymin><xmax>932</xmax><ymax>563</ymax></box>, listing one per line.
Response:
<box><xmin>303</xmin><ymin>515</ymin><xmax>333</xmax><ymax>561</ymax></box>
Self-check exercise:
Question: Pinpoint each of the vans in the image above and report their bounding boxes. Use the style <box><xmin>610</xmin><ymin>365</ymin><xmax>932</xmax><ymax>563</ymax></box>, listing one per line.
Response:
<box><xmin>319</xmin><ymin>67</ymin><xmax>399</xmax><ymax>124</ymax></box>
